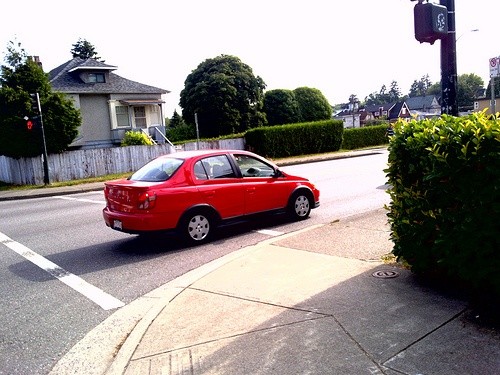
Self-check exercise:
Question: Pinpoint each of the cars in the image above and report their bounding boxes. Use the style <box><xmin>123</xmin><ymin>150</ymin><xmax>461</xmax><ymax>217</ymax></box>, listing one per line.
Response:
<box><xmin>103</xmin><ymin>149</ymin><xmax>321</xmax><ymax>246</ymax></box>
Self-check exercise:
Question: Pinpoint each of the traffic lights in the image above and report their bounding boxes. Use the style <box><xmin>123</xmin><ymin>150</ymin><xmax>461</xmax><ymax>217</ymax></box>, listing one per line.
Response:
<box><xmin>26</xmin><ymin>92</ymin><xmax>44</xmax><ymax>131</ymax></box>
